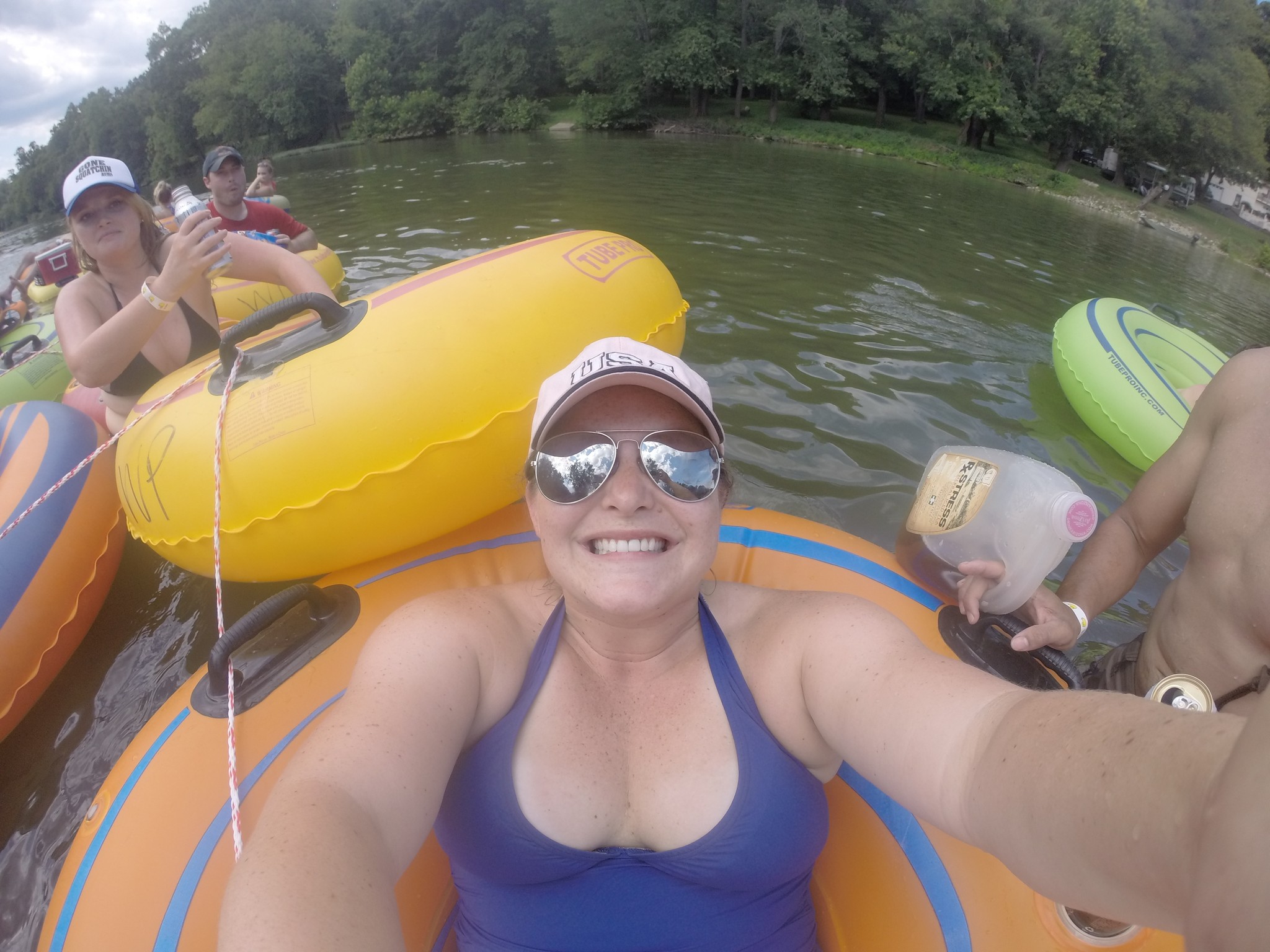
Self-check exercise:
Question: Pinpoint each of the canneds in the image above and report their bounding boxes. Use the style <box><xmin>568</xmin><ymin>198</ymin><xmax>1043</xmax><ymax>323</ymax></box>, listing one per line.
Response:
<box><xmin>1144</xmin><ymin>674</ymin><xmax>1218</xmax><ymax>713</ymax></box>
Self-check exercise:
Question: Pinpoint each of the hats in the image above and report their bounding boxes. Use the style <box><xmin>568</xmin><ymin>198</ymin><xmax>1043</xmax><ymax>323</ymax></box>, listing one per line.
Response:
<box><xmin>202</xmin><ymin>145</ymin><xmax>245</xmax><ymax>177</ymax></box>
<box><xmin>527</xmin><ymin>335</ymin><xmax>726</xmax><ymax>459</ymax></box>
<box><xmin>63</xmin><ymin>155</ymin><xmax>137</xmax><ymax>217</ymax></box>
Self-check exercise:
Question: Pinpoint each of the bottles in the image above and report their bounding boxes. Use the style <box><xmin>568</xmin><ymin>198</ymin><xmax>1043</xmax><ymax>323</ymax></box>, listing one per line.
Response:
<box><xmin>904</xmin><ymin>445</ymin><xmax>1098</xmax><ymax>616</ymax></box>
<box><xmin>171</xmin><ymin>184</ymin><xmax>234</xmax><ymax>280</ymax></box>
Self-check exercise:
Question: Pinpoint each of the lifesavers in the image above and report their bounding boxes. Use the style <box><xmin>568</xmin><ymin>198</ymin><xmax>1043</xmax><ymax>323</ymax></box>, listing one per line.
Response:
<box><xmin>210</xmin><ymin>240</ymin><xmax>349</xmax><ymax>332</ymax></box>
<box><xmin>27</xmin><ymin>258</ymin><xmax>92</xmax><ymax>303</ymax></box>
<box><xmin>1049</xmin><ymin>295</ymin><xmax>1233</xmax><ymax>474</ymax></box>
<box><xmin>20</xmin><ymin>263</ymin><xmax>36</xmax><ymax>283</ymax></box>
<box><xmin>0</xmin><ymin>312</ymin><xmax>75</xmax><ymax>412</ymax></box>
<box><xmin>114</xmin><ymin>228</ymin><xmax>690</xmax><ymax>585</ymax></box>
<box><xmin>242</xmin><ymin>194</ymin><xmax>291</xmax><ymax>214</ymax></box>
<box><xmin>0</xmin><ymin>400</ymin><xmax>127</xmax><ymax>744</ymax></box>
<box><xmin>36</xmin><ymin>499</ymin><xmax>1198</xmax><ymax>952</ymax></box>
<box><xmin>153</xmin><ymin>215</ymin><xmax>178</xmax><ymax>236</ymax></box>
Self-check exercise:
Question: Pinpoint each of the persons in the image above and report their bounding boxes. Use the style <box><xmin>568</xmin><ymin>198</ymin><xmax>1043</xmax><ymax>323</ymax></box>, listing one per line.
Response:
<box><xmin>54</xmin><ymin>156</ymin><xmax>340</xmax><ymax>439</ymax></box>
<box><xmin>214</xmin><ymin>337</ymin><xmax>1270</xmax><ymax>952</ymax></box>
<box><xmin>246</xmin><ymin>158</ymin><xmax>279</xmax><ymax>198</ymax></box>
<box><xmin>0</xmin><ymin>233</ymin><xmax>85</xmax><ymax>301</ymax></box>
<box><xmin>954</xmin><ymin>345</ymin><xmax>1270</xmax><ymax>720</ymax></box>
<box><xmin>151</xmin><ymin>180</ymin><xmax>180</xmax><ymax>220</ymax></box>
<box><xmin>198</xmin><ymin>146</ymin><xmax>319</xmax><ymax>254</ymax></box>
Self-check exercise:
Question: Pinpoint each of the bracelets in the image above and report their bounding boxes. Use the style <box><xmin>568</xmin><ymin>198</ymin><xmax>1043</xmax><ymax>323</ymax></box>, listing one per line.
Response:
<box><xmin>1056</xmin><ymin>594</ymin><xmax>1091</xmax><ymax>642</ymax></box>
<box><xmin>141</xmin><ymin>275</ymin><xmax>178</xmax><ymax>311</ymax></box>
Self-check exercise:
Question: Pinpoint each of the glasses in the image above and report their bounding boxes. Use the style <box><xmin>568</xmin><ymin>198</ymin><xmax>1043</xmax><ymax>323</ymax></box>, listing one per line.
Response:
<box><xmin>530</xmin><ymin>430</ymin><xmax>726</xmax><ymax>505</ymax></box>
